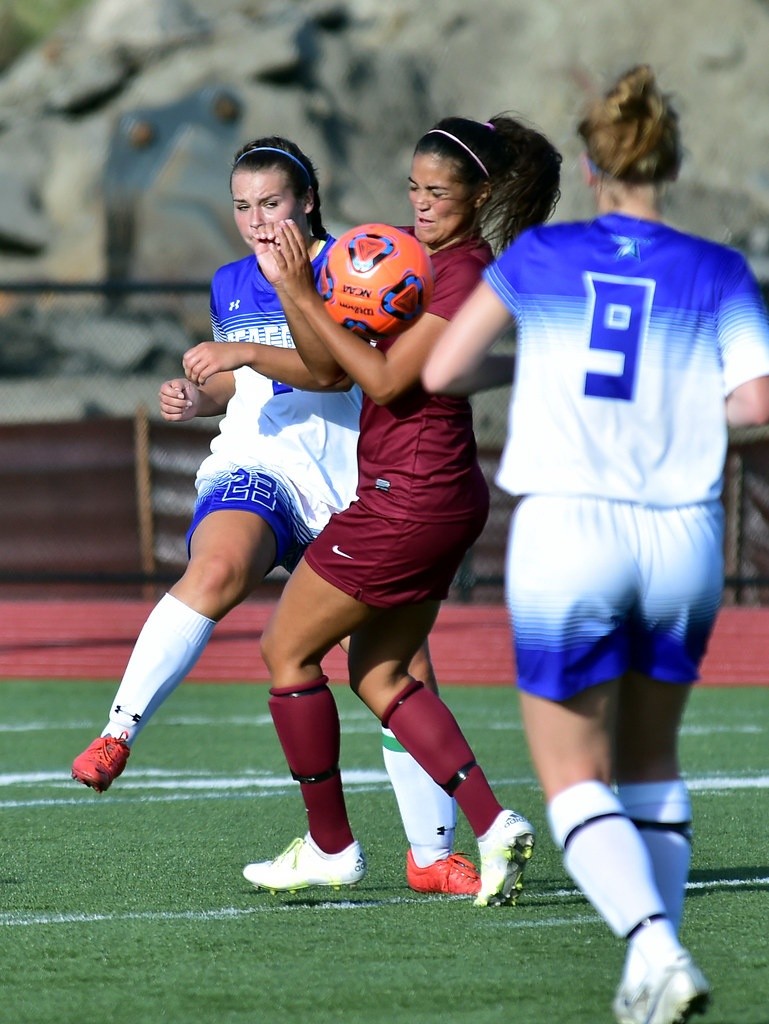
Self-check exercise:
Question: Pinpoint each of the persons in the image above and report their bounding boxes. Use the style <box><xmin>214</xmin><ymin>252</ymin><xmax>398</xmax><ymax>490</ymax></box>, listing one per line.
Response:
<box><xmin>420</xmin><ymin>65</ymin><xmax>769</xmax><ymax>1024</ymax></box>
<box><xmin>67</xmin><ymin>135</ymin><xmax>492</xmax><ymax>901</ymax></box>
<box><xmin>244</xmin><ymin>110</ymin><xmax>564</xmax><ymax>915</ymax></box>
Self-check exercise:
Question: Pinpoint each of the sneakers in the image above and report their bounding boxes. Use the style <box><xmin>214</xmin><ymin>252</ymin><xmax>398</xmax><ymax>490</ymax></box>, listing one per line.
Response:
<box><xmin>471</xmin><ymin>809</ymin><xmax>536</xmax><ymax>909</ymax></box>
<box><xmin>404</xmin><ymin>849</ymin><xmax>482</xmax><ymax>896</ymax></box>
<box><xmin>242</xmin><ymin>838</ymin><xmax>368</xmax><ymax>895</ymax></box>
<box><xmin>70</xmin><ymin>735</ymin><xmax>131</xmax><ymax>793</ymax></box>
<box><xmin>612</xmin><ymin>944</ymin><xmax>711</xmax><ymax>1024</ymax></box>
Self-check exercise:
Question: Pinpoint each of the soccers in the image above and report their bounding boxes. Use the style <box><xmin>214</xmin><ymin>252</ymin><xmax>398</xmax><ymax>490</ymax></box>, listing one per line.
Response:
<box><xmin>320</xmin><ymin>224</ymin><xmax>433</xmax><ymax>339</ymax></box>
